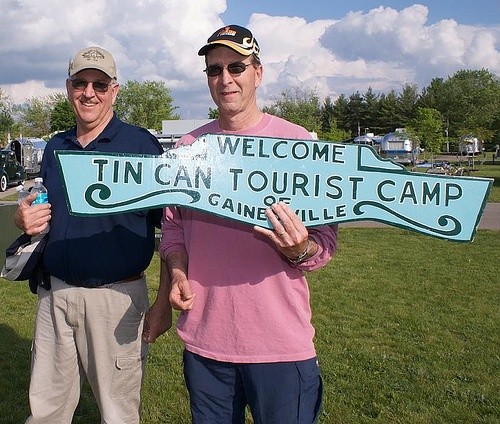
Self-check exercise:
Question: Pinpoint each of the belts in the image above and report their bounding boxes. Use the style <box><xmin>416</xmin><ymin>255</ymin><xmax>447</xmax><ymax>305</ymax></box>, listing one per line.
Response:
<box><xmin>65</xmin><ymin>272</ymin><xmax>146</xmax><ymax>288</ymax></box>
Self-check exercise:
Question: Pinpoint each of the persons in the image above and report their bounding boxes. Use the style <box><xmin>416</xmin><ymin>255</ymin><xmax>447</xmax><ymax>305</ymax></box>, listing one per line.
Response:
<box><xmin>12</xmin><ymin>44</ymin><xmax>175</xmax><ymax>424</ymax></box>
<box><xmin>155</xmin><ymin>23</ymin><xmax>343</xmax><ymax>424</ymax></box>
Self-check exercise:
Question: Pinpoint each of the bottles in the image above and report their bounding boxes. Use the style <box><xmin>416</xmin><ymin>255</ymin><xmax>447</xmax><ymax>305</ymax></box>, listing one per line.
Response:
<box><xmin>31</xmin><ymin>178</ymin><xmax>50</xmax><ymax>236</ymax></box>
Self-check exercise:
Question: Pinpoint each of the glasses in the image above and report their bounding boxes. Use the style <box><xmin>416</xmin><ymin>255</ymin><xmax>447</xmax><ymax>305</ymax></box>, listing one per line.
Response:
<box><xmin>203</xmin><ymin>61</ymin><xmax>257</xmax><ymax>76</ymax></box>
<box><xmin>69</xmin><ymin>79</ymin><xmax>115</xmax><ymax>92</ymax></box>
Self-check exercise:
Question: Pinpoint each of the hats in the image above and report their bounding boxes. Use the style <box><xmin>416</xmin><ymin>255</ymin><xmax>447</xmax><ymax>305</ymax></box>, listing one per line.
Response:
<box><xmin>198</xmin><ymin>25</ymin><xmax>262</xmax><ymax>63</ymax></box>
<box><xmin>68</xmin><ymin>47</ymin><xmax>118</xmax><ymax>81</ymax></box>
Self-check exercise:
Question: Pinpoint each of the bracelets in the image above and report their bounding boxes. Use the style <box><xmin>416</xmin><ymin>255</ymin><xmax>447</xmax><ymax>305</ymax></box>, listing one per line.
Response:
<box><xmin>288</xmin><ymin>240</ymin><xmax>311</xmax><ymax>264</ymax></box>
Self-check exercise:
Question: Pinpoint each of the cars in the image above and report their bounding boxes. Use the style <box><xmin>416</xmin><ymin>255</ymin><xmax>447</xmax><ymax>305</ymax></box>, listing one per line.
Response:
<box><xmin>0</xmin><ymin>149</ymin><xmax>27</xmax><ymax>192</ymax></box>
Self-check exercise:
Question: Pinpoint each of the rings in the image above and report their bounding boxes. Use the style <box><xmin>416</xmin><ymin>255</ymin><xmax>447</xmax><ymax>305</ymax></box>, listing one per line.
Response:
<box><xmin>278</xmin><ymin>230</ymin><xmax>286</xmax><ymax>237</ymax></box>
